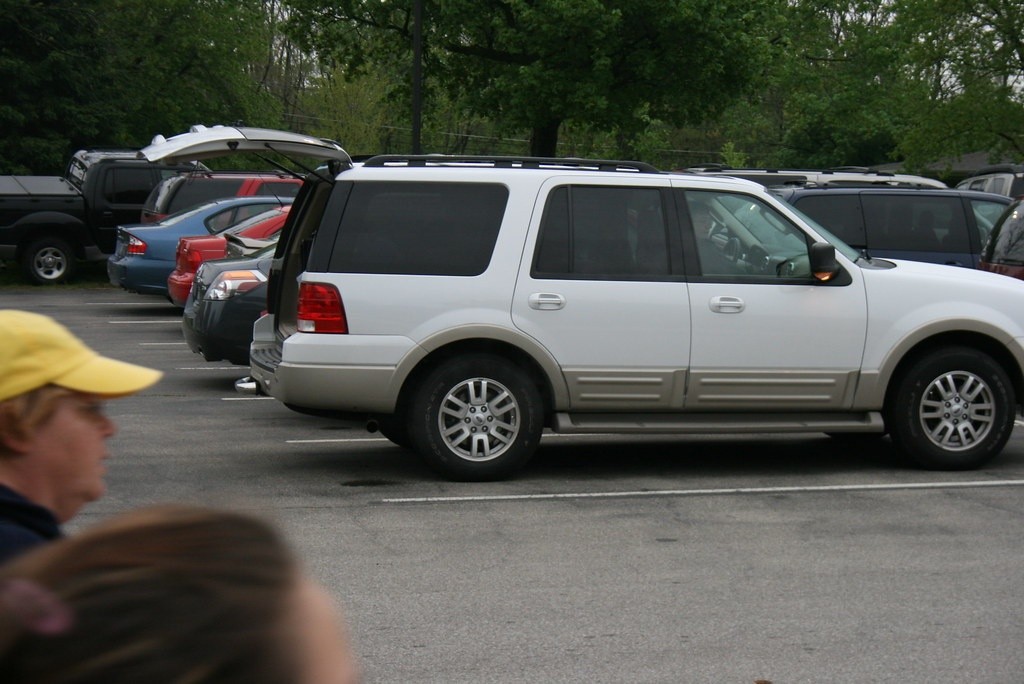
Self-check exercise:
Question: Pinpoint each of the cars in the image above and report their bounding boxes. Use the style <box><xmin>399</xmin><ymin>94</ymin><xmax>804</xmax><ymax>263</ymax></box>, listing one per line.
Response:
<box><xmin>688</xmin><ymin>164</ymin><xmax>1024</xmax><ymax>281</ymax></box>
<box><xmin>168</xmin><ymin>207</ymin><xmax>295</xmax><ymax>368</ymax></box>
<box><xmin>104</xmin><ymin>197</ymin><xmax>303</xmax><ymax>301</ymax></box>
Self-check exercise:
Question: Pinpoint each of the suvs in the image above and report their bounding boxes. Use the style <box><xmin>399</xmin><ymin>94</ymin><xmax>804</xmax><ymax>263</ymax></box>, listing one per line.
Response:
<box><xmin>134</xmin><ymin>122</ymin><xmax>1024</xmax><ymax>483</ymax></box>
<box><xmin>141</xmin><ymin>169</ymin><xmax>311</xmax><ymax>226</ymax></box>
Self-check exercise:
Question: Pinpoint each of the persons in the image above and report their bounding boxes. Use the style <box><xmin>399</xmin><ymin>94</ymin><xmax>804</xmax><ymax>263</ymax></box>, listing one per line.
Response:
<box><xmin>0</xmin><ymin>308</ymin><xmax>165</xmax><ymax>572</ymax></box>
<box><xmin>687</xmin><ymin>200</ymin><xmax>747</xmax><ymax>275</ymax></box>
<box><xmin>0</xmin><ymin>500</ymin><xmax>359</xmax><ymax>684</ymax></box>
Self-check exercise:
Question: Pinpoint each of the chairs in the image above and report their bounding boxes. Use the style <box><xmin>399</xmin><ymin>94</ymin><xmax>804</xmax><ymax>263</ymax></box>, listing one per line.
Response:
<box><xmin>633</xmin><ymin>203</ymin><xmax>669</xmax><ymax>275</ymax></box>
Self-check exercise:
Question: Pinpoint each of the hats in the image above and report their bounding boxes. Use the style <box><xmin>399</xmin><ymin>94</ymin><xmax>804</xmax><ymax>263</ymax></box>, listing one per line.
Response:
<box><xmin>0</xmin><ymin>309</ymin><xmax>164</xmax><ymax>404</ymax></box>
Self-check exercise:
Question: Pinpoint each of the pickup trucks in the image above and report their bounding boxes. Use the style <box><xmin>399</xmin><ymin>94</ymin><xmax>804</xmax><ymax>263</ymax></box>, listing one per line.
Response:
<box><xmin>0</xmin><ymin>150</ymin><xmax>217</xmax><ymax>288</ymax></box>
